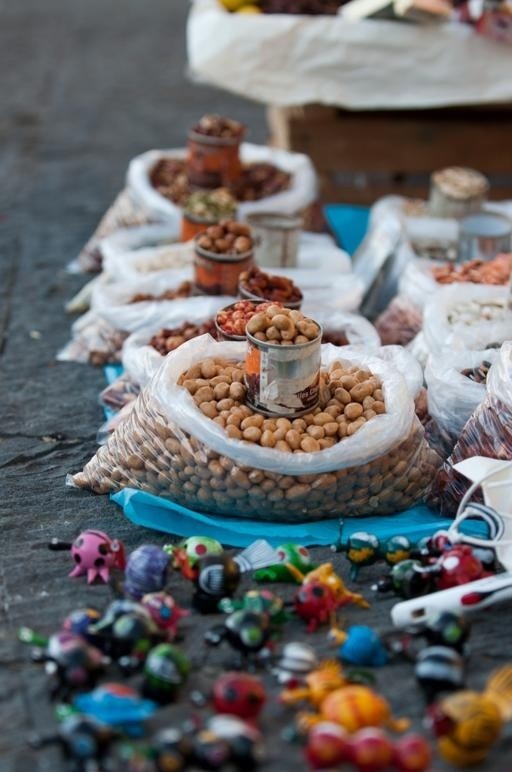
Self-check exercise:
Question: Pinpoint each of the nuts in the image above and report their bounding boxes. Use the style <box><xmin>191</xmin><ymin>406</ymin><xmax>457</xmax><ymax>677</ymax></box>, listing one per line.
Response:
<box><xmin>352</xmin><ymin>165</ymin><xmax>512</xmax><ymax>520</ymax></box>
<box><xmin>64</xmin><ymin>116</ymin><xmax>442</xmax><ymax>519</ymax></box>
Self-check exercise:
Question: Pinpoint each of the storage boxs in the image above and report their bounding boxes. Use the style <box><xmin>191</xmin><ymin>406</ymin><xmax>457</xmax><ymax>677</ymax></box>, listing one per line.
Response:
<box><xmin>268</xmin><ymin>103</ymin><xmax>512</xmax><ymax>234</ymax></box>
